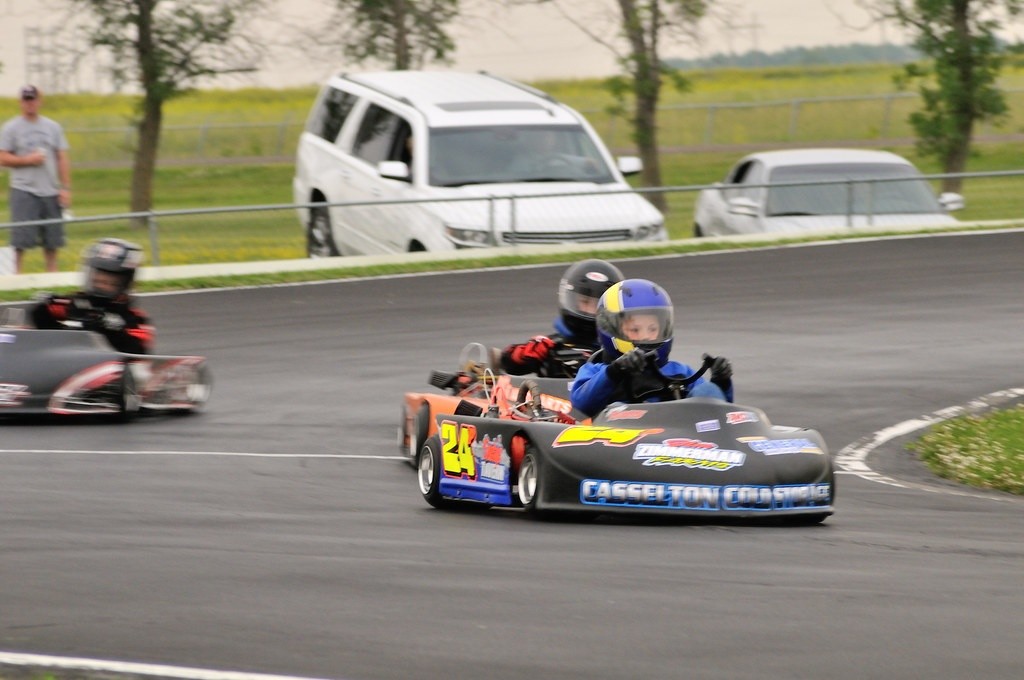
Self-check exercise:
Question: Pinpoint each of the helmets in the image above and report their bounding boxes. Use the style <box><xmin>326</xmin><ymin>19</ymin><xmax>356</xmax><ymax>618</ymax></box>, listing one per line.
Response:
<box><xmin>86</xmin><ymin>238</ymin><xmax>143</xmax><ymax>307</ymax></box>
<box><xmin>558</xmin><ymin>259</ymin><xmax>624</xmax><ymax>339</ymax></box>
<box><xmin>595</xmin><ymin>279</ymin><xmax>674</xmax><ymax>369</ymax></box>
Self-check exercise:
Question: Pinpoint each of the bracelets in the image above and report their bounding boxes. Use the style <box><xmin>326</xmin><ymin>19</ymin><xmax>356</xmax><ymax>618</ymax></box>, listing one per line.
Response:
<box><xmin>62</xmin><ymin>185</ymin><xmax>73</xmax><ymax>192</ymax></box>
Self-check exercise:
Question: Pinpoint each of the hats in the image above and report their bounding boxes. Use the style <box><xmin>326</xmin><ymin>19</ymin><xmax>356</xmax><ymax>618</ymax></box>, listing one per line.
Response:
<box><xmin>22</xmin><ymin>85</ymin><xmax>37</xmax><ymax>99</ymax></box>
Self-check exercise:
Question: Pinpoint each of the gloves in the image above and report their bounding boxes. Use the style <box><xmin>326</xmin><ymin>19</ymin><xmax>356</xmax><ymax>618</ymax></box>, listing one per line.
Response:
<box><xmin>612</xmin><ymin>349</ymin><xmax>648</xmax><ymax>382</ymax></box>
<box><xmin>702</xmin><ymin>356</ymin><xmax>733</xmax><ymax>383</ymax></box>
<box><xmin>511</xmin><ymin>336</ymin><xmax>556</xmax><ymax>364</ymax></box>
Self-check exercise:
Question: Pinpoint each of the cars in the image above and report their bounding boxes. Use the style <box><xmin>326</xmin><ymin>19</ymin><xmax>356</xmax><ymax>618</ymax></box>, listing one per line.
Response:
<box><xmin>690</xmin><ymin>148</ymin><xmax>967</xmax><ymax>241</ymax></box>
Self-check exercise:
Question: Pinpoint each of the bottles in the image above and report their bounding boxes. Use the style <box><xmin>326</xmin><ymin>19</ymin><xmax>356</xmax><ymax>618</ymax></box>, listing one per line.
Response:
<box><xmin>56</xmin><ymin>193</ymin><xmax>76</xmax><ymax>221</ymax></box>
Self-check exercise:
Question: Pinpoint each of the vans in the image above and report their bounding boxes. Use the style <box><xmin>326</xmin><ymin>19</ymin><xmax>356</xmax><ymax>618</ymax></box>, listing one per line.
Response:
<box><xmin>291</xmin><ymin>67</ymin><xmax>667</xmax><ymax>261</ymax></box>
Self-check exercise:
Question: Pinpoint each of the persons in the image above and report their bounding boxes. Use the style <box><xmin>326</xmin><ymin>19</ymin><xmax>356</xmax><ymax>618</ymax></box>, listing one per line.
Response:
<box><xmin>30</xmin><ymin>236</ymin><xmax>155</xmax><ymax>354</ymax></box>
<box><xmin>502</xmin><ymin>259</ymin><xmax>734</xmax><ymax>410</ymax></box>
<box><xmin>0</xmin><ymin>85</ymin><xmax>72</xmax><ymax>275</ymax></box>
